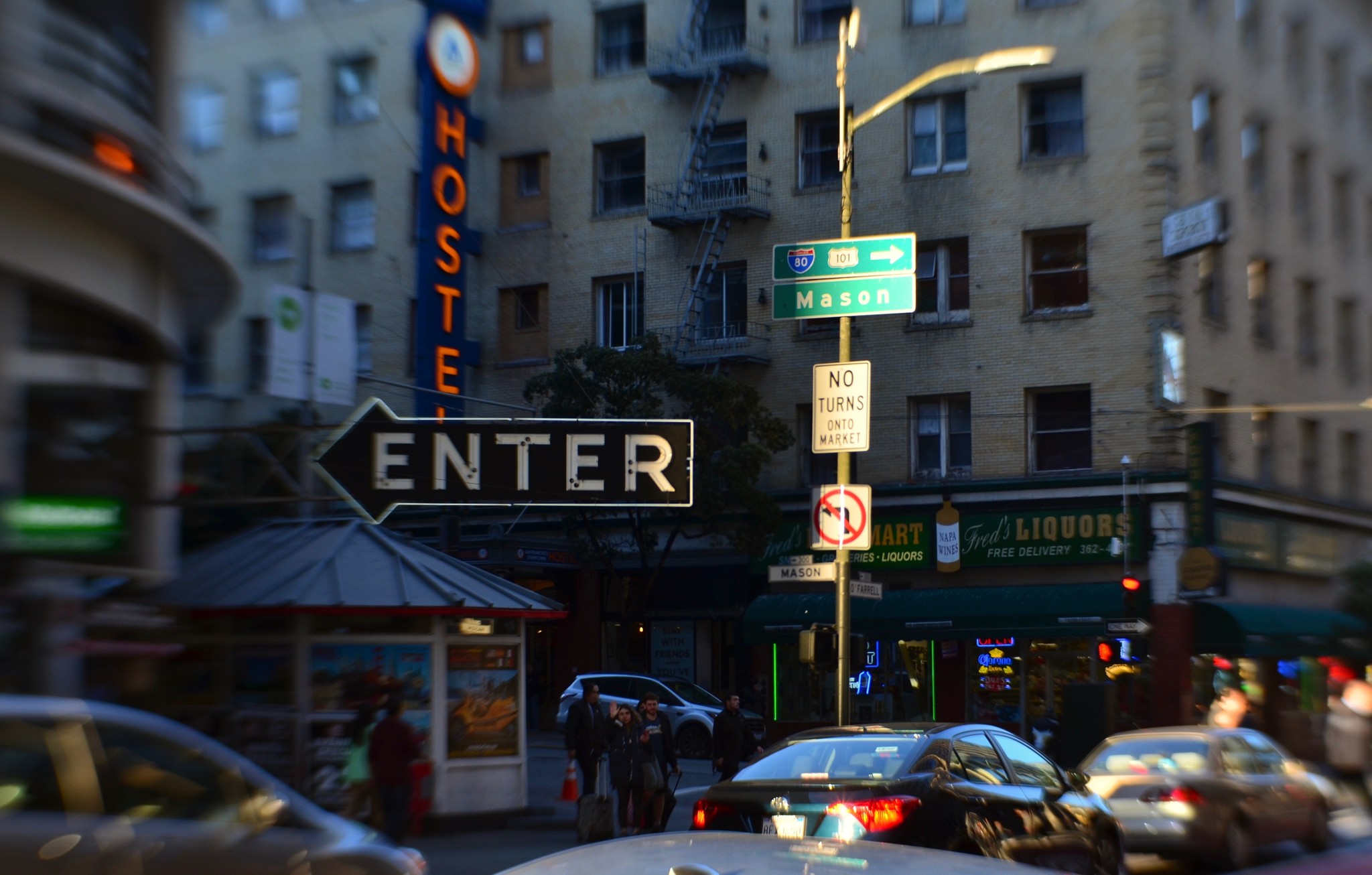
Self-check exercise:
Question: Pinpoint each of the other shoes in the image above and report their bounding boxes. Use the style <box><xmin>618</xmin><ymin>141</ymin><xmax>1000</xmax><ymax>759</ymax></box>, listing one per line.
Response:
<box><xmin>633</xmin><ymin>827</ymin><xmax>641</xmax><ymax>835</ymax></box>
<box><xmin>619</xmin><ymin>828</ymin><xmax>628</xmax><ymax>837</ymax></box>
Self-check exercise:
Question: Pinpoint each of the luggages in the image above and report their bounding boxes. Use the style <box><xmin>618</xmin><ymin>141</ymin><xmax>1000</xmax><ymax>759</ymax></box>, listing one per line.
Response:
<box><xmin>643</xmin><ymin>771</ymin><xmax>682</xmax><ymax>831</ymax></box>
<box><xmin>578</xmin><ymin>756</ymin><xmax>617</xmax><ymax>845</ymax></box>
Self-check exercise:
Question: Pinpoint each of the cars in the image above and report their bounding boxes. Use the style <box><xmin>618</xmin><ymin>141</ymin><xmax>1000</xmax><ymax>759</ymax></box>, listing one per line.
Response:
<box><xmin>1078</xmin><ymin>726</ymin><xmax>1339</xmax><ymax>869</ymax></box>
<box><xmin>690</xmin><ymin>723</ymin><xmax>1127</xmax><ymax>875</ymax></box>
<box><xmin>555</xmin><ymin>672</ymin><xmax>724</xmax><ymax>760</ymax></box>
<box><xmin>0</xmin><ymin>692</ymin><xmax>431</xmax><ymax>875</ymax></box>
<box><xmin>497</xmin><ymin>831</ymin><xmax>1058</xmax><ymax>875</ymax></box>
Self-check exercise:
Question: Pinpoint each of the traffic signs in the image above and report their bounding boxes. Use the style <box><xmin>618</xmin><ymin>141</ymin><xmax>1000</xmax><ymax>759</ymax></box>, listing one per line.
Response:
<box><xmin>1105</xmin><ymin>618</ymin><xmax>1151</xmax><ymax>638</ymax></box>
<box><xmin>303</xmin><ymin>394</ymin><xmax>695</xmax><ymax>522</ymax></box>
<box><xmin>768</xmin><ymin>231</ymin><xmax>925</xmax><ymax>325</ymax></box>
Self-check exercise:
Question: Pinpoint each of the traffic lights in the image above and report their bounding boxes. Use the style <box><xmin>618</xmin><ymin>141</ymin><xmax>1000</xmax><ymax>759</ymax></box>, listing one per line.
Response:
<box><xmin>798</xmin><ymin>629</ymin><xmax>831</xmax><ymax>668</ymax></box>
<box><xmin>1119</xmin><ymin>575</ymin><xmax>1151</xmax><ymax>617</ymax></box>
<box><xmin>1094</xmin><ymin>639</ymin><xmax>1123</xmax><ymax>685</ymax></box>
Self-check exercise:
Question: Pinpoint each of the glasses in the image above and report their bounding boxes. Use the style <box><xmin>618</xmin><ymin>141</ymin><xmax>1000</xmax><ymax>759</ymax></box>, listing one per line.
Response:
<box><xmin>594</xmin><ymin>691</ymin><xmax>600</xmax><ymax>695</ymax></box>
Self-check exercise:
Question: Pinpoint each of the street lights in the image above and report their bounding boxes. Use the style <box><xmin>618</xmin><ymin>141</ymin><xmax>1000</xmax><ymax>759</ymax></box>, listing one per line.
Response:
<box><xmin>825</xmin><ymin>12</ymin><xmax>1054</xmax><ymax>723</ymax></box>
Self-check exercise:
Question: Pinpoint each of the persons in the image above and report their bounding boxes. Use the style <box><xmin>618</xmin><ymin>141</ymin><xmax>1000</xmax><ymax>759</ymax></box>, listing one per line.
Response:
<box><xmin>526</xmin><ymin>664</ymin><xmax>540</xmax><ymax>732</ymax></box>
<box><xmin>604</xmin><ymin>700</ymin><xmax>650</xmax><ymax>835</ymax></box>
<box><xmin>638</xmin><ymin>693</ymin><xmax>680</xmax><ymax>832</ymax></box>
<box><xmin>344</xmin><ymin>704</ymin><xmax>382</xmax><ymax>830</ymax></box>
<box><xmin>744</xmin><ymin>685</ymin><xmax>763</xmax><ymax>716</ymax></box>
<box><xmin>566</xmin><ymin>683</ymin><xmax>605</xmax><ymax>804</ymax></box>
<box><xmin>406</xmin><ymin>732</ymin><xmax>434</xmax><ymax>842</ymax></box>
<box><xmin>369</xmin><ymin>697</ymin><xmax>426</xmax><ymax>843</ymax></box>
<box><xmin>712</xmin><ymin>693</ymin><xmax>764</xmax><ymax>783</ymax></box>
<box><xmin>1031</xmin><ymin>707</ymin><xmax>1059</xmax><ymax>761</ymax></box>
<box><xmin>1323</xmin><ymin>693</ymin><xmax>1371</xmax><ymax>793</ymax></box>
<box><xmin>636</xmin><ymin>700</ymin><xmax>646</xmax><ymax>713</ymax></box>
<box><xmin>1207</xmin><ymin>670</ymin><xmax>1247</xmax><ymax>726</ymax></box>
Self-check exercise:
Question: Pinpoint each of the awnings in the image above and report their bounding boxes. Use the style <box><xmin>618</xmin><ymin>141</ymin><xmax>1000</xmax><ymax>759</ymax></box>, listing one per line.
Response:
<box><xmin>1188</xmin><ymin>601</ymin><xmax>1372</xmax><ymax>665</ymax></box>
<box><xmin>603</xmin><ymin>565</ymin><xmax>770</xmax><ymax>622</ymax></box>
<box><xmin>741</xmin><ymin>580</ymin><xmax>1156</xmax><ymax>638</ymax></box>
